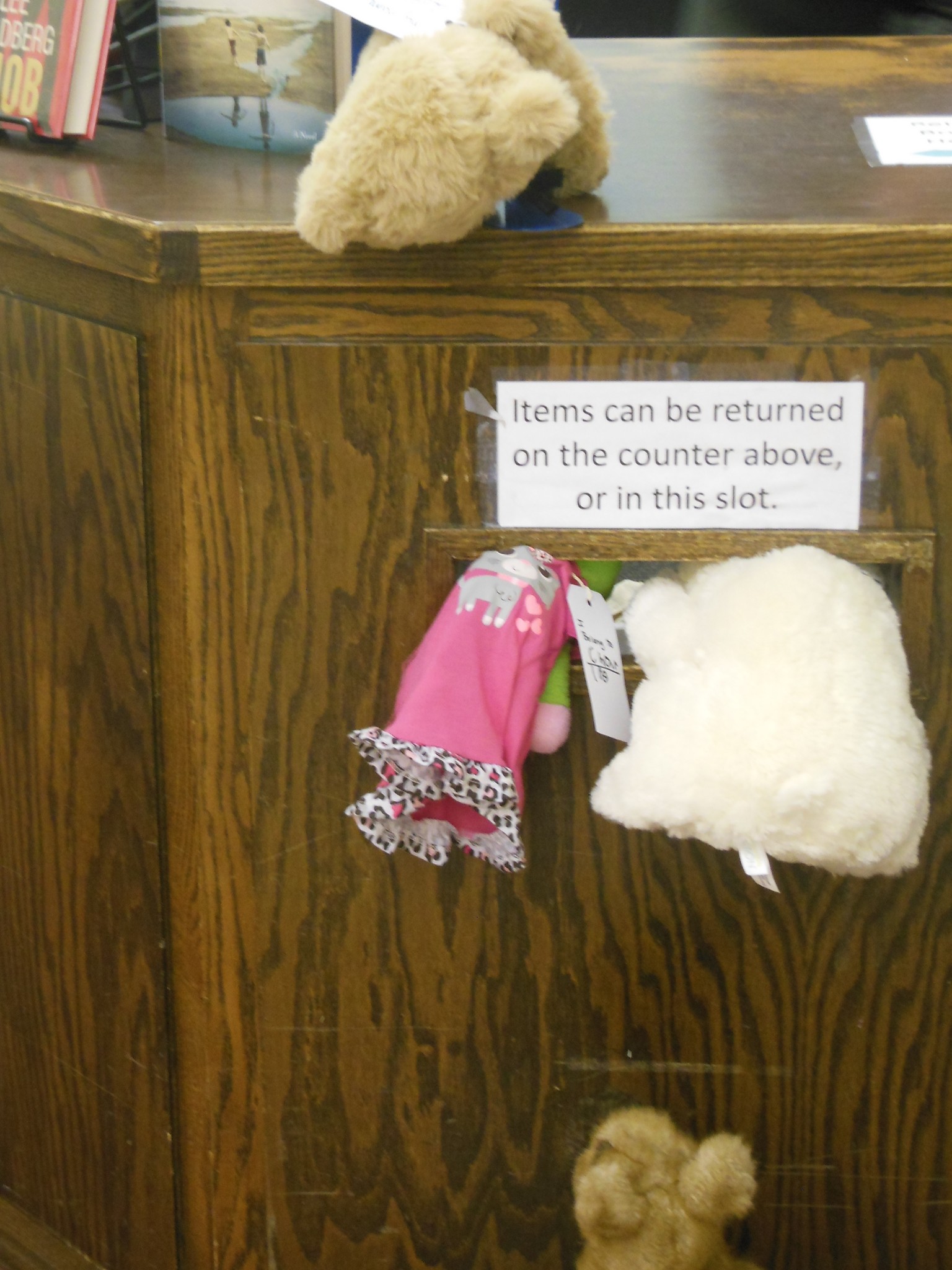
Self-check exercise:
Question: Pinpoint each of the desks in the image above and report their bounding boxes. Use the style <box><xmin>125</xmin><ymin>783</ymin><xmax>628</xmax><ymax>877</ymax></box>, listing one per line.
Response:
<box><xmin>0</xmin><ymin>30</ymin><xmax>952</xmax><ymax>1270</ymax></box>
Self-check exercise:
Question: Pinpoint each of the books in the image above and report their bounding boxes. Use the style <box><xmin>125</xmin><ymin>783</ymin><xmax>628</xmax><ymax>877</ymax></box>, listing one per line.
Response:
<box><xmin>0</xmin><ymin>0</ymin><xmax>119</xmax><ymax>140</ymax></box>
<box><xmin>156</xmin><ymin>1</ymin><xmax>352</xmax><ymax>163</ymax></box>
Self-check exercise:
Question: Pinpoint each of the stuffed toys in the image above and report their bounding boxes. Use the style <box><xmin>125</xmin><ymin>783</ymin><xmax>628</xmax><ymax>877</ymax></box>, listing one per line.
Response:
<box><xmin>557</xmin><ymin>1102</ymin><xmax>758</xmax><ymax>1270</ymax></box>
<box><xmin>289</xmin><ymin>0</ymin><xmax>607</xmax><ymax>252</ymax></box>
